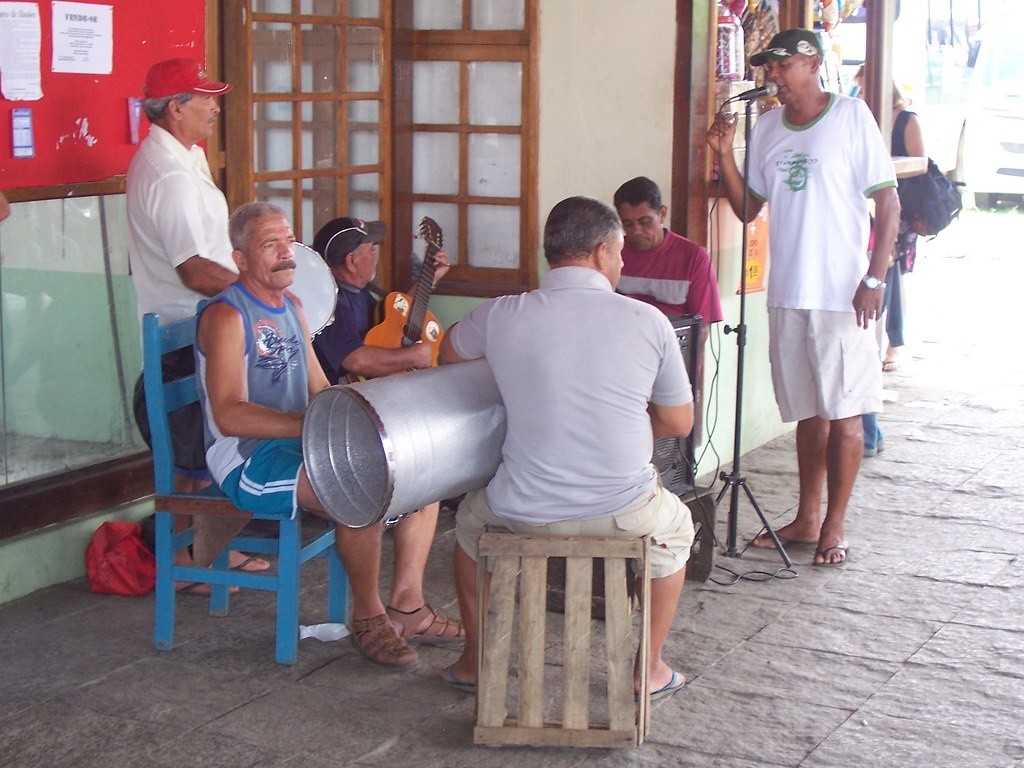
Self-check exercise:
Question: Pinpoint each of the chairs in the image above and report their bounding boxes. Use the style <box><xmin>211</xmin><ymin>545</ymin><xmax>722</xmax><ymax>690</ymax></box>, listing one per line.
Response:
<box><xmin>143</xmin><ymin>299</ymin><xmax>349</xmax><ymax>663</ymax></box>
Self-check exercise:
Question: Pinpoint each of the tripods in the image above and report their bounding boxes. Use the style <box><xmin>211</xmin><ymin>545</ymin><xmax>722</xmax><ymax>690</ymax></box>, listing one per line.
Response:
<box><xmin>714</xmin><ymin>97</ymin><xmax>795</xmax><ymax>567</ymax></box>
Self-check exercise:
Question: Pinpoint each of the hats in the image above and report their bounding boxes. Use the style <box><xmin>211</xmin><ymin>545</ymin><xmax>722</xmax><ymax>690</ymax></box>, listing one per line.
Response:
<box><xmin>750</xmin><ymin>28</ymin><xmax>823</xmax><ymax>67</ymax></box>
<box><xmin>313</xmin><ymin>217</ymin><xmax>386</xmax><ymax>265</ymax></box>
<box><xmin>144</xmin><ymin>58</ymin><xmax>233</xmax><ymax>98</ymax></box>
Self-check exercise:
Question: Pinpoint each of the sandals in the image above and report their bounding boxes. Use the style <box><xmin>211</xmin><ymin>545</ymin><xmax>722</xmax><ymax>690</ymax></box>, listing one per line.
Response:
<box><xmin>386</xmin><ymin>604</ymin><xmax>466</xmax><ymax>645</ymax></box>
<box><xmin>349</xmin><ymin>612</ymin><xmax>422</xmax><ymax>669</ymax></box>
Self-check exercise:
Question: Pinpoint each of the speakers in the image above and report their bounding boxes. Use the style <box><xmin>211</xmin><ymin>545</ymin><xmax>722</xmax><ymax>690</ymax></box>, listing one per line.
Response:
<box><xmin>646</xmin><ymin>313</ymin><xmax>703</xmax><ymax>498</ymax></box>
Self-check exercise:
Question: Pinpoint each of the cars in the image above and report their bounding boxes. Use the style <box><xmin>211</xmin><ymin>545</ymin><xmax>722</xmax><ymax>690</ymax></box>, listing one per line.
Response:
<box><xmin>815</xmin><ymin>0</ymin><xmax>1023</xmax><ymax>219</ymax></box>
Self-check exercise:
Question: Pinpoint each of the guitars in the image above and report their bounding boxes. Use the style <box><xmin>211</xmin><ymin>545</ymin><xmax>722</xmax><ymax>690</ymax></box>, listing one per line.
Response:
<box><xmin>351</xmin><ymin>214</ymin><xmax>444</xmax><ymax>380</ymax></box>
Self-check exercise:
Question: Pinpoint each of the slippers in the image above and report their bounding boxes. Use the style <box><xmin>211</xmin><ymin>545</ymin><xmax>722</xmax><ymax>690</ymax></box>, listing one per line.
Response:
<box><xmin>882</xmin><ymin>361</ymin><xmax>897</xmax><ymax>371</ymax></box>
<box><xmin>751</xmin><ymin>531</ymin><xmax>819</xmax><ymax>549</ymax></box>
<box><xmin>229</xmin><ymin>556</ymin><xmax>271</xmax><ymax>572</ymax></box>
<box><xmin>175</xmin><ymin>583</ymin><xmax>213</xmax><ymax>596</ymax></box>
<box><xmin>635</xmin><ymin>670</ymin><xmax>685</xmax><ymax>701</ymax></box>
<box><xmin>813</xmin><ymin>544</ymin><xmax>849</xmax><ymax>568</ymax></box>
<box><xmin>441</xmin><ymin>665</ymin><xmax>476</xmax><ymax>693</ymax></box>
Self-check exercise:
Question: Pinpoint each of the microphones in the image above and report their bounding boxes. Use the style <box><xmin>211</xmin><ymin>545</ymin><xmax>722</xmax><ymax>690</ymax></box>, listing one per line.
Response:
<box><xmin>724</xmin><ymin>82</ymin><xmax>778</xmax><ymax>105</ymax></box>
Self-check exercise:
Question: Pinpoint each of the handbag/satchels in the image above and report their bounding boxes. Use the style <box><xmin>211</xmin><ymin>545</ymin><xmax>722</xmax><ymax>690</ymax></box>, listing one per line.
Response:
<box><xmin>892</xmin><ymin>110</ymin><xmax>964</xmax><ymax>236</ymax></box>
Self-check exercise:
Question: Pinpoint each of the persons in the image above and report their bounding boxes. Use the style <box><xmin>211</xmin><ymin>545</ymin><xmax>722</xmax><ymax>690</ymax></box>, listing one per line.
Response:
<box><xmin>309</xmin><ymin>216</ymin><xmax>452</xmax><ymax>378</ymax></box>
<box><xmin>852</xmin><ymin>60</ymin><xmax>929</xmax><ymax>373</ymax></box>
<box><xmin>124</xmin><ymin>57</ymin><xmax>271</xmax><ymax>598</ymax></box>
<box><xmin>438</xmin><ymin>196</ymin><xmax>696</xmax><ymax>701</ymax></box>
<box><xmin>613</xmin><ymin>178</ymin><xmax>722</xmax><ymax>328</ymax></box>
<box><xmin>704</xmin><ymin>29</ymin><xmax>902</xmax><ymax>567</ymax></box>
<box><xmin>196</xmin><ymin>201</ymin><xmax>467</xmax><ymax>665</ymax></box>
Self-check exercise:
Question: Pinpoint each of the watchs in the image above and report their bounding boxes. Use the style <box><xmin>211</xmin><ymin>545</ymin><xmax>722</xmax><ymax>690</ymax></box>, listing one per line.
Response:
<box><xmin>861</xmin><ymin>273</ymin><xmax>888</xmax><ymax>291</ymax></box>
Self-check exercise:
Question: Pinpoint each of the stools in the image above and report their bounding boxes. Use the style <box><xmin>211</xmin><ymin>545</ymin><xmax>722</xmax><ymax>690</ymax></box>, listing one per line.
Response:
<box><xmin>472</xmin><ymin>524</ymin><xmax>651</xmax><ymax>750</ymax></box>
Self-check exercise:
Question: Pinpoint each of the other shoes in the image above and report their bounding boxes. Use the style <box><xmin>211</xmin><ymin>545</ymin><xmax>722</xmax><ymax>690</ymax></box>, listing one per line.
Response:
<box><xmin>876</xmin><ymin>442</ymin><xmax>885</xmax><ymax>452</ymax></box>
<box><xmin>864</xmin><ymin>448</ymin><xmax>877</xmax><ymax>457</ymax></box>
